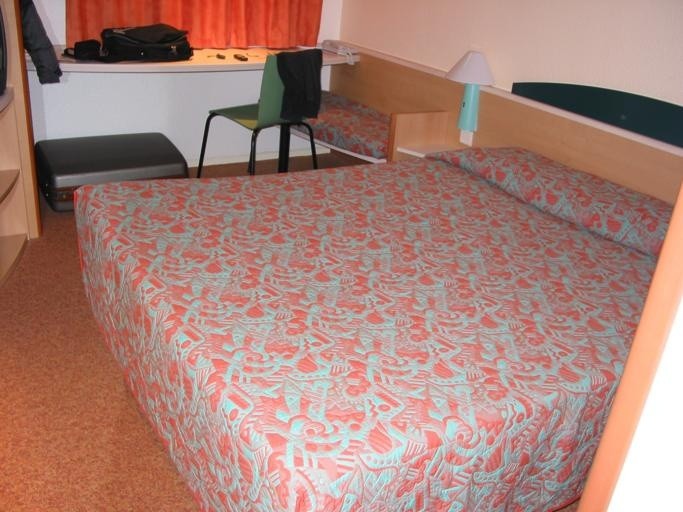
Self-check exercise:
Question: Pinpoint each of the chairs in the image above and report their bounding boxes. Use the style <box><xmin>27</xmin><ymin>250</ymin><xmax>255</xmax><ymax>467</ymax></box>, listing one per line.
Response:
<box><xmin>195</xmin><ymin>50</ymin><xmax>322</xmax><ymax>177</ymax></box>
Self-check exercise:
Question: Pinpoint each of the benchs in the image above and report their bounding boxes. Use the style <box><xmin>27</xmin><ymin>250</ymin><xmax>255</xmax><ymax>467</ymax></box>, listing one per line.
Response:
<box><xmin>291</xmin><ymin>88</ymin><xmax>392</xmax><ymax>164</ymax></box>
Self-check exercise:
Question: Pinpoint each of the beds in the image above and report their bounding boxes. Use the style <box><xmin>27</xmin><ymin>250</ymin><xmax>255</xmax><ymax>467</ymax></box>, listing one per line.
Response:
<box><xmin>71</xmin><ymin>145</ymin><xmax>675</xmax><ymax>512</ymax></box>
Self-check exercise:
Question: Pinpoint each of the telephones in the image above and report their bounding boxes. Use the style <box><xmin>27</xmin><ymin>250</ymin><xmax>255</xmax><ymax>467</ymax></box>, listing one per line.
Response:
<box><xmin>321</xmin><ymin>39</ymin><xmax>360</xmax><ymax>55</ymax></box>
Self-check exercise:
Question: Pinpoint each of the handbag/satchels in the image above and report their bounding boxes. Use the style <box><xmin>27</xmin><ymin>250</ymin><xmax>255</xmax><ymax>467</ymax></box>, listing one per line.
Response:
<box><xmin>101</xmin><ymin>24</ymin><xmax>193</xmax><ymax>62</ymax></box>
<box><xmin>74</xmin><ymin>39</ymin><xmax>100</xmax><ymax>59</ymax></box>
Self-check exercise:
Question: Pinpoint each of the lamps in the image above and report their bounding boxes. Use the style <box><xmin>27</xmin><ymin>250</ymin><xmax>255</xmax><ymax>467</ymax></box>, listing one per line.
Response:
<box><xmin>445</xmin><ymin>49</ymin><xmax>492</xmax><ymax>132</ymax></box>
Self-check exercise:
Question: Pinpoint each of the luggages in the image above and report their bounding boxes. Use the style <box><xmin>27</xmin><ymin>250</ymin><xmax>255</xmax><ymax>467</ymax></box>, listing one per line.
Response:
<box><xmin>34</xmin><ymin>133</ymin><xmax>189</xmax><ymax>212</ymax></box>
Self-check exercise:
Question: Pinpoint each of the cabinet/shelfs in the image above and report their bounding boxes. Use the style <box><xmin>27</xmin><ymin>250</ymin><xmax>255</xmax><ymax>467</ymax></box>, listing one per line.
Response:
<box><xmin>1</xmin><ymin>0</ymin><xmax>43</xmax><ymax>290</ymax></box>
